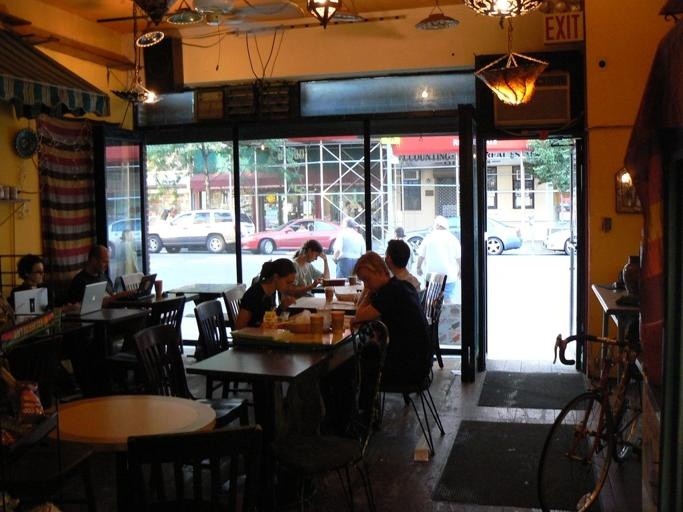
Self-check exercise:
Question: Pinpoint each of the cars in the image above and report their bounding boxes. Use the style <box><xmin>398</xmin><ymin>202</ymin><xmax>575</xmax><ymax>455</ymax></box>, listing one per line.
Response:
<box><xmin>394</xmin><ymin>212</ymin><xmax>524</xmax><ymax>257</ymax></box>
<box><xmin>239</xmin><ymin>217</ymin><xmax>344</xmax><ymax>255</ymax></box>
<box><xmin>108</xmin><ymin>217</ymin><xmax>142</xmax><ymax>259</ymax></box>
<box><xmin>541</xmin><ymin>223</ymin><xmax>578</xmax><ymax>254</ymax></box>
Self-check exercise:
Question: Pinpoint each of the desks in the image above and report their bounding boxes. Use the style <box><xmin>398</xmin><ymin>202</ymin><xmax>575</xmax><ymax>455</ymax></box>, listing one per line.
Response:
<box><xmin>591</xmin><ymin>284</ymin><xmax>640</xmax><ymax>386</ymax></box>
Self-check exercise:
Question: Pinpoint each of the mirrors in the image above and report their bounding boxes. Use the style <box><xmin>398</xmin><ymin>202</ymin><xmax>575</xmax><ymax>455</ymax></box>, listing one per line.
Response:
<box><xmin>615</xmin><ymin>167</ymin><xmax>643</xmax><ymax>214</ymax></box>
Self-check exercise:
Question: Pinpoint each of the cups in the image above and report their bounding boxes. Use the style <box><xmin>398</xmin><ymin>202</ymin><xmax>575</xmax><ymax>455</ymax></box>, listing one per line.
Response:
<box><xmin>52</xmin><ymin>308</ymin><xmax>63</xmax><ymax>329</ymax></box>
<box><xmin>154</xmin><ymin>280</ymin><xmax>161</xmax><ymax>297</ymax></box>
<box><xmin>323</xmin><ymin>287</ymin><xmax>334</xmax><ymax>302</ymax></box>
<box><xmin>310</xmin><ymin>313</ymin><xmax>324</xmax><ymax>337</ymax></box>
<box><xmin>349</xmin><ymin>278</ymin><xmax>356</xmax><ymax>286</ymax></box>
<box><xmin>330</xmin><ymin>311</ymin><xmax>345</xmax><ymax>332</ymax></box>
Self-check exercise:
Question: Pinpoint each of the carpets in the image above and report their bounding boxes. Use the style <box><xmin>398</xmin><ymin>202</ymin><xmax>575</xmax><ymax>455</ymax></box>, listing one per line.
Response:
<box><xmin>477</xmin><ymin>370</ymin><xmax>589</xmax><ymax>411</ymax></box>
<box><xmin>430</xmin><ymin>420</ymin><xmax>603</xmax><ymax>512</ymax></box>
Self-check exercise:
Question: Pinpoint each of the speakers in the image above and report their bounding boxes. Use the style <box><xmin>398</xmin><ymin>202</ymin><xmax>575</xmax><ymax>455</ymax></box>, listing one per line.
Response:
<box><xmin>144</xmin><ymin>37</ymin><xmax>184</xmax><ymax>93</ymax></box>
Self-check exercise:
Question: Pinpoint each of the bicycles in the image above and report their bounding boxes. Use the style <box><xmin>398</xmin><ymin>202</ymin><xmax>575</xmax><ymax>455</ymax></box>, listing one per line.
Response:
<box><xmin>537</xmin><ymin>314</ymin><xmax>639</xmax><ymax>512</ymax></box>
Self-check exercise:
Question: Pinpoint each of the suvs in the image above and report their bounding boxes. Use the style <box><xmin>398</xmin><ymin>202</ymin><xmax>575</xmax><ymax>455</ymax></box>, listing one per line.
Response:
<box><xmin>148</xmin><ymin>207</ymin><xmax>254</xmax><ymax>255</ymax></box>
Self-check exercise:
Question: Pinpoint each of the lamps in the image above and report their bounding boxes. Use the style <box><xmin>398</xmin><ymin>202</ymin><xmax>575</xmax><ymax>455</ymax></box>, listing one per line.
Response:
<box><xmin>464</xmin><ymin>0</ymin><xmax>550</xmax><ymax>112</ymax></box>
<box><xmin>136</xmin><ymin>0</ymin><xmax>364</xmax><ymax>47</ymax></box>
<box><xmin>416</xmin><ymin>1</ymin><xmax>459</xmax><ymax>33</ymax></box>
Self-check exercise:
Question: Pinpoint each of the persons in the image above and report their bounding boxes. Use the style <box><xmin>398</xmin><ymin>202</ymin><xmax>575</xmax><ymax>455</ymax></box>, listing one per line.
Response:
<box><xmin>115</xmin><ymin>227</ymin><xmax>141</xmax><ymax>278</ymax></box>
<box><xmin>382</xmin><ymin>238</ymin><xmax>421</xmax><ymax>292</ymax></box>
<box><xmin>415</xmin><ymin>214</ymin><xmax>461</xmax><ymax>306</ymax></box>
<box><xmin>5</xmin><ymin>252</ymin><xmax>57</xmax><ymax>316</ymax></box>
<box><xmin>353</xmin><ymin>201</ymin><xmax>366</xmax><ymax>233</ymax></box>
<box><xmin>327</xmin><ymin>250</ymin><xmax>436</xmax><ymax>440</ymax></box>
<box><xmin>342</xmin><ymin>200</ymin><xmax>351</xmax><ymax>216</ymax></box>
<box><xmin>289</xmin><ymin>238</ymin><xmax>332</xmax><ymax>300</ymax></box>
<box><xmin>331</xmin><ymin>216</ymin><xmax>368</xmax><ymax>278</ymax></box>
<box><xmin>232</xmin><ymin>257</ymin><xmax>298</xmax><ymax>332</ymax></box>
<box><xmin>392</xmin><ymin>225</ymin><xmax>415</xmax><ymax>274</ymax></box>
<box><xmin>67</xmin><ymin>242</ymin><xmax>116</xmax><ymax>307</ymax></box>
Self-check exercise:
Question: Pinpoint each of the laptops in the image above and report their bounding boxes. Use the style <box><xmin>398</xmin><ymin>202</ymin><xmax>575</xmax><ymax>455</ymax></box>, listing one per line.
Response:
<box><xmin>65</xmin><ymin>280</ymin><xmax>108</xmax><ymax>315</ymax></box>
<box><xmin>135</xmin><ymin>274</ymin><xmax>157</xmax><ymax>299</ymax></box>
